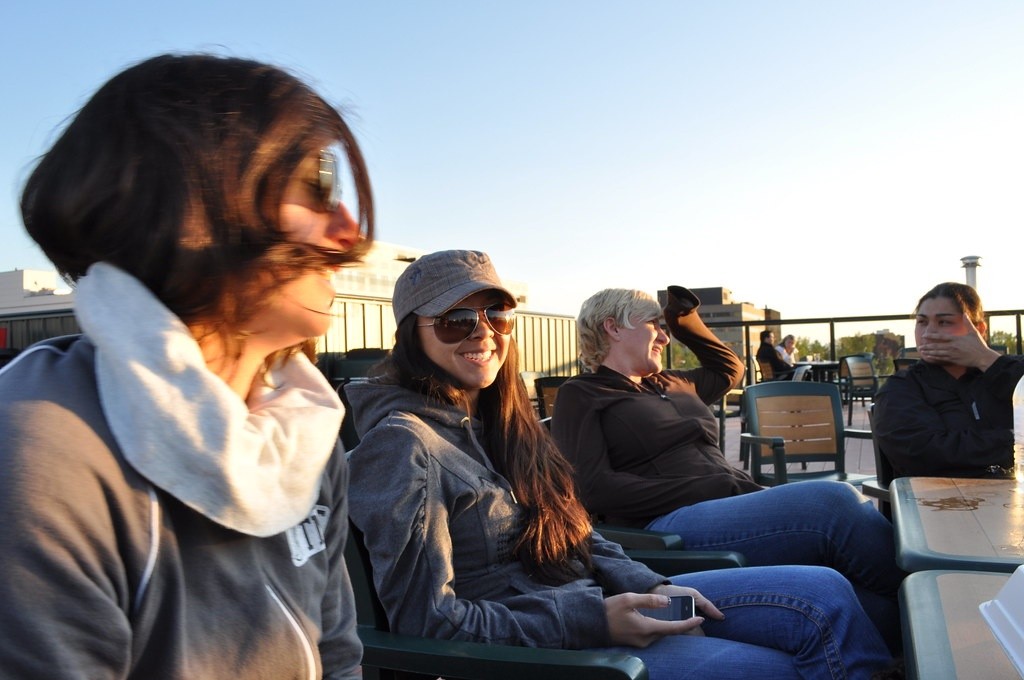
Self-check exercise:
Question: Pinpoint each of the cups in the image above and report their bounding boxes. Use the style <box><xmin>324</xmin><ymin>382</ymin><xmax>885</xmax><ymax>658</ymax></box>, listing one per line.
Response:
<box><xmin>814</xmin><ymin>353</ymin><xmax>820</xmax><ymax>361</ymax></box>
<box><xmin>806</xmin><ymin>355</ymin><xmax>813</xmax><ymax>362</ymax></box>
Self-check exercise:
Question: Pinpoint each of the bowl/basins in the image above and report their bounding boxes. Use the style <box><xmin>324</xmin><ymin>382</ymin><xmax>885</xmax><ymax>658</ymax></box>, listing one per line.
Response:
<box><xmin>979</xmin><ymin>564</ymin><xmax>1023</xmax><ymax>679</ymax></box>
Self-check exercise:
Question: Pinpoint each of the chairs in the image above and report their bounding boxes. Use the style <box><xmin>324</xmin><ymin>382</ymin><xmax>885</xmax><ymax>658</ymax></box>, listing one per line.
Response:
<box><xmin>738</xmin><ymin>353</ymin><xmax>920</xmax><ymax>490</ymax></box>
<box><xmin>330</xmin><ymin>376</ymin><xmax>747</xmax><ymax>680</ymax></box>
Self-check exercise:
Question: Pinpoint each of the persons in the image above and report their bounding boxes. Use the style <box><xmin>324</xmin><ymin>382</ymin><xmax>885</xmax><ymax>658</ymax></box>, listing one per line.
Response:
<box><xmin>776</xmin><ymin>334</ymin><xmax>799</xmax><ymax>367</ymax></box>
<box><xmin>874</xmin><ymin>282</ymin><xmax>1024</xmax><ymax>518</ymax></box>
<box><xmin>0</xmin><ymin>53</ymin><xmax>375</xmax><ymax>680</ymax></box>
<box><xmin>550</xmin><ymin>285</ymin><xmax>904</xmax><ymax>654</ymax></box>
<box><xmin>756</xmin><ymin>330</ymin><xmax>811</xmax><ymax>381</ymax></box>
<box><xmin>346</xmin><ymin>250</ymin><xmax>906</xmax><ymax>680</ymax></box>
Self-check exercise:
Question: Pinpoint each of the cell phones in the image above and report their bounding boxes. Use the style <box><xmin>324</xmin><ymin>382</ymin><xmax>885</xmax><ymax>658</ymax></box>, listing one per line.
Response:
<box><xmin>636</xmin><ymin>595</ymin><xmax>695</xmax><ymax>621</ymax></box>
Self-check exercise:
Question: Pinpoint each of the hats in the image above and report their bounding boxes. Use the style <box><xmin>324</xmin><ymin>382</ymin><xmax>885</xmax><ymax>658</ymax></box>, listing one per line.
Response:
<box><xmin>392</xmin><ymin>250</ymin><xmax>517</xmax><ymax>329</ymax></box>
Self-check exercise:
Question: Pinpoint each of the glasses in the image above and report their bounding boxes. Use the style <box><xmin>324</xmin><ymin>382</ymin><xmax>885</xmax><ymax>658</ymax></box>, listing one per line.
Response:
<box><xmin>416</xmin><ymin>302</ymin><xmax>516</xmax><ymax>344</ymax></box>
<box><xmin>265</xmin><ymin>149</ymin><xmax>341</xmax><ymax>212</ymax></box>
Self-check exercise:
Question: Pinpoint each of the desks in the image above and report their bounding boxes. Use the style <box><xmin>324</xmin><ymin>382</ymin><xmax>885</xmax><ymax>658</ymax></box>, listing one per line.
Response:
<box><xmin>792</xmin><ymin>361</ymin><xmax>841</xmax><ymax>382</ymax></box>
<box><xmin>888</xmin><ymin>474</ymin><xmax>1024</xmax><ymax>680</ymax></box>
